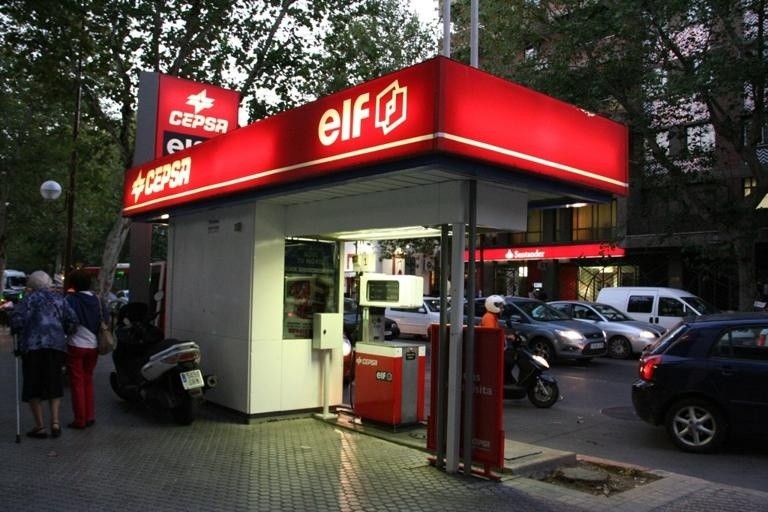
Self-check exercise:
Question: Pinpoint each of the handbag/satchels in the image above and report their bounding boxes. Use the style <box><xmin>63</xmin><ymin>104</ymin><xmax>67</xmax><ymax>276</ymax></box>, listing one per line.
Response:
<box><xmin>98</xmin><ymin>321</ymin><xmax>114</xmax><ymax>354</ymax></box>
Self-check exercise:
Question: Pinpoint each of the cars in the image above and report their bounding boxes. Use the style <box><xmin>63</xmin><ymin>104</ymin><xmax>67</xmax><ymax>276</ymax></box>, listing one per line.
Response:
<box><xmin>531</xmin><ymin>298</ymin><xmax>668</xmax><ymax>361</ymax></box>
<box><xmin>629</xmin><ymin>311</ymin><xmax>768</xmax><ymax>454</ymax></box>
<box><xmin>342</xmin><ymin>296</ymin><xmax>401</xmax><ymax>347</ymax></box>
<box><xmin>444</xmin><ymin>295</ymin><xmax>609</xmax><ymax>368</ymax></box>
<box><xmin>383</xmin><ymin>297</ymin><xmax>452</xmax><ymax>342</ymax></box>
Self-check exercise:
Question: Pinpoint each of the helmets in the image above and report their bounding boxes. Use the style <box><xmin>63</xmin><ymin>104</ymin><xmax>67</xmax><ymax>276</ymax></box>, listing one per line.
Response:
<box><xmin>484</xmin><ymin>294</ymin><xmax>506</xmax><ymax>314</ymax></box>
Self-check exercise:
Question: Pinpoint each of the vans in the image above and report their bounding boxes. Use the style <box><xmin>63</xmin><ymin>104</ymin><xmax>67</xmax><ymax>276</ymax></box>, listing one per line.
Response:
<box><xmin>595</xmin><ymin>285</ymin><xmax>727</xmax><ymax>331</ymax></box>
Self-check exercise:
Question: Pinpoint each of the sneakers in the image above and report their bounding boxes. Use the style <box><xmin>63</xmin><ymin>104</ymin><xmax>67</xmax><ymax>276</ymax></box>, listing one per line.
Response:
<box><xmin>66</xmin><ymin>417</ymin><xmax>95</xmax><ymax>429</ymax></box>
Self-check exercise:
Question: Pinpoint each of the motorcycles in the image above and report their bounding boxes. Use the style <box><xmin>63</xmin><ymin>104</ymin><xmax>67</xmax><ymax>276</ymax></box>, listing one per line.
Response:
<box><xmin>107</xmin><ymin>286</ymin><xmax>219</xmax><ymax>425</ymax></box>
<box><xmin>503</xmin><ymin>331</ymin><xmax>560</xmax><ymax>410</ymax></box>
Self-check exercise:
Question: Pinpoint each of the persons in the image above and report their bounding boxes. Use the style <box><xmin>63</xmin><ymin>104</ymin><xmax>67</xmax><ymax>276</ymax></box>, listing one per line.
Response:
<box><xmin>10</xmin><ymin>270</ymin><xmax>80</xmax><ymax>439</ymax></box>
<box><xmin>540</xmin><ymin>288</ymin><xmax>548</xmax><ymax>301</ymax></box>
<box><xmin>527</xmin><ymin>286</ymin><xmax>536</xmax><ymax>299</ymax></box>
<box><xmin>478</xmin><ymin>294</ymin><xmax>517</xmax><ymax>385</ymax></box>
<box><xmin>63</xmin><ymin>269</ymin><xmax>109</xmax><ymax>429</ymax></box>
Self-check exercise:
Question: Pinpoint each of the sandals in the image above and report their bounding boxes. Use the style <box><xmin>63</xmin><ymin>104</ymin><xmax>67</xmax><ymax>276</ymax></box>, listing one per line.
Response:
<box><xmin>26</xmin><ymin>426</ymin><xmax>47</xmax><ymax>439</ymax></box>
<box><xmin>51</xmin><ymin>422</ymin><xmax>61</xmax><ymax>437</ymax></box>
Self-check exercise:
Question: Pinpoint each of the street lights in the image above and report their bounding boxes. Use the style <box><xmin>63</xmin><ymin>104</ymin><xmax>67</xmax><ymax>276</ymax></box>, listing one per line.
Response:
<box><xmin>41</xmin><ymin>179</ymin><xmax>76</xmax><ymax>298</ymax></box>
